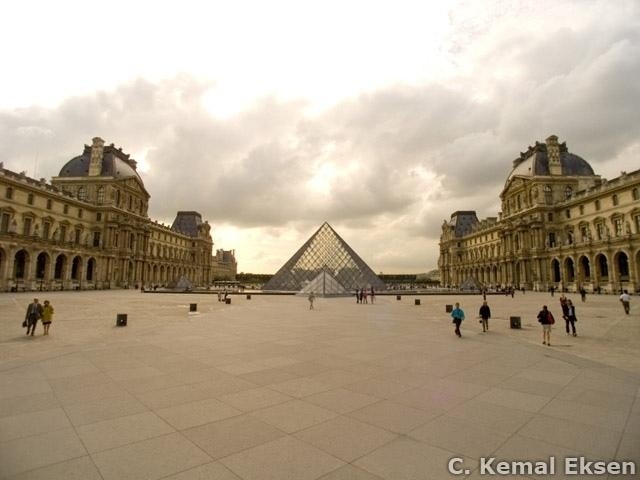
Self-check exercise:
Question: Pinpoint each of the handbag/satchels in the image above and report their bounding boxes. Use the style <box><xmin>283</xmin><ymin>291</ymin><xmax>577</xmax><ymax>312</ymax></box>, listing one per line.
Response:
<box><xmin>23</xmin><ymin>321</ymin><xmax>27</xmax><ymax>327</ymax></box>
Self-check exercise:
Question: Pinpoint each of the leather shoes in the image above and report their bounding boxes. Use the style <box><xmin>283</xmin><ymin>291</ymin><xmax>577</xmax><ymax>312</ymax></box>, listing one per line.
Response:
<box><xmin>27</xmin><ymin>332</ymin><xmax>30</xmax><ymax>334</ymax></box>
<box><xmin>31</xmin><ymin>333</ymin><xmax>34</xmax><ymax>336</ymax></box>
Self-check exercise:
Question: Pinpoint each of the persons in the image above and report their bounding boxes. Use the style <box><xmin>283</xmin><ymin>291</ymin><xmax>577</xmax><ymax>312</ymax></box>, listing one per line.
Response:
<box><xmin>562</xmin><ymin>299</ymin><xmax>579</xmax><ymax>338</ymax></box>
<box><xmin>559</xmin><ymin>293</ymin><xmax>568</xmax><ymax>316</ymax></box>
<box><xmin>308</xmin><ymin>291</ymin><xmax>317</xmax><ymax>310</ymax></box>
<box><xmin>40</xmin><ymin>300</ymin><xmax>55</xmax><ymax>336</ymax></box>
<box><xmin>369</xmin><ymin>286</ymin><xmax>375</xmax><ymax>304</ymax></box>
<box><xmin>355</xmin><ymin>288</ymin><xmax>359</xmax><ymax>304</ymax></box>
<box><xmin>23</xmin><ymin>297</ymin><xmax>44</xmax><ymax>337</ymax></box>
<box><xmin>619</xmin><ymin>289</ymin><xmax>633</xmax><ymax>315</ymax></box>
<box><xmin>479</xmin><ymin>300</ymin><xmax>491</xmax><ymax>333</ymax></box>
<box><xmin>536</xmin><ymin>304</ymin><xmax>555</xmax><ymax>346</ymax></box>
<box><xmin>450</xmin><ymin>302</ymin><xmax>466</xmax><ymax>338</ymax></box>
<box><xmin>580</xmin><ymin>287</ymin><xmax>587</xmax><ymax>302</ymax></box>
<box><xmin>363</xmin><ymin>289</ymin><xmax>369</xmax><ymax>304</ymax></box>
<box><xmin>359</xmin><ymin>288</ymin><xmax>363</xmax><ymax>304</ymax></box>
